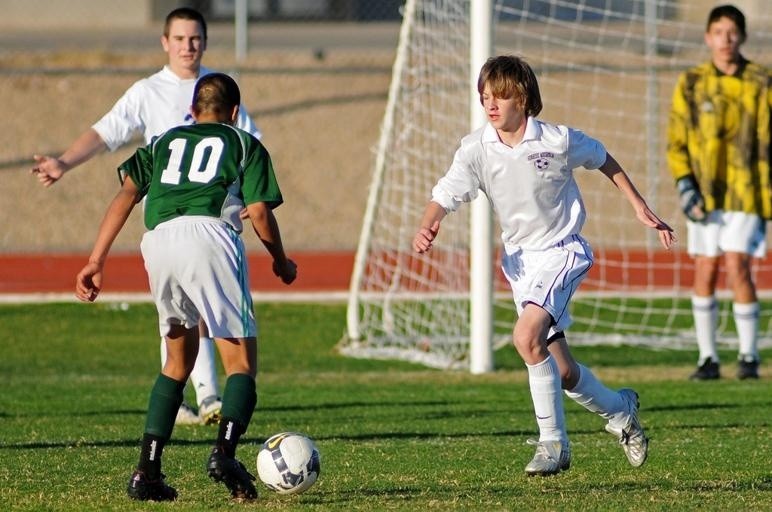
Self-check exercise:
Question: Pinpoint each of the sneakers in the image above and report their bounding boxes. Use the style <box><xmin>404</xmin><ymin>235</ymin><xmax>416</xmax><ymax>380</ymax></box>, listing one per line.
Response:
<box><xmin>196</xmin><ymin>399</ymin><xmax>227</xmax><ymax>428</ymax></box>
<box><xmin>731</xmin><ymin>353</ymin><xmax>760</xmax><ymax>380</ymax></box>
<box><xmin>172</xmin><ymin>402</ymin><xmax>203</xmax><ymax>426</ymax></box>
<box><xmin>125</xmin><ymin>468</ymin><xmax>183</xmax><ymax>503</ymax></box>
<box><xmin>688</xmin><ymin>356</ymin><xmax>720</xmax><ymax>382</ymax></box>
<box><xmin>520</xmin><ymin>437</ymin><xmax>573</xmax><ymax>476</ymax></box>
<box><xmin>205</xmin><ymin>448</ymin><xmax>260</xmax><ymax>500</ymax></box>
<box><xmin>601</xmin><ymin>385</ymin><xmax>649</xmax><ymax>470</ymax></box>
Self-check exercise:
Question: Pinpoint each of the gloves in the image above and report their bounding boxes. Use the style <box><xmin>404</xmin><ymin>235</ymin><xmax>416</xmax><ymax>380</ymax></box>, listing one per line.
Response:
<box><xmin>674</xmin><ymin>176</ymin><xmax>709</xmax><ymax>224</ymax></box>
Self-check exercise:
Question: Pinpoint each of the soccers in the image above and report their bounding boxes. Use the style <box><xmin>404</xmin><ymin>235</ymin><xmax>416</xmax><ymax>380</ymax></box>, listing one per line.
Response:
<box><xmin>257</xmin><ymin>432</ymin><xmax>320</xmax><ymax>494</ymax></box>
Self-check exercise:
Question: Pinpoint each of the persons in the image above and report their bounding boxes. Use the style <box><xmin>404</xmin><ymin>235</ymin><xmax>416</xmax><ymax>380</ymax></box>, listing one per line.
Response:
<box><xmin>78</xmin><ymin>73</ymin><xmax>299</xmax><ymax>502</ymax></box>
<box><xmin>28</xmin><ymin>7</ymin><xmax>263</xmax><ymax>426</ymax></box>
<box><xmin>663</xmin><ymin>4</ymin><xmax>772</xmax><ymax>380</ymax></box>
<box><xmin>411</xmin><ymin>55</ymin><xmax>678</xmax><ymax>476</ymax></box>
